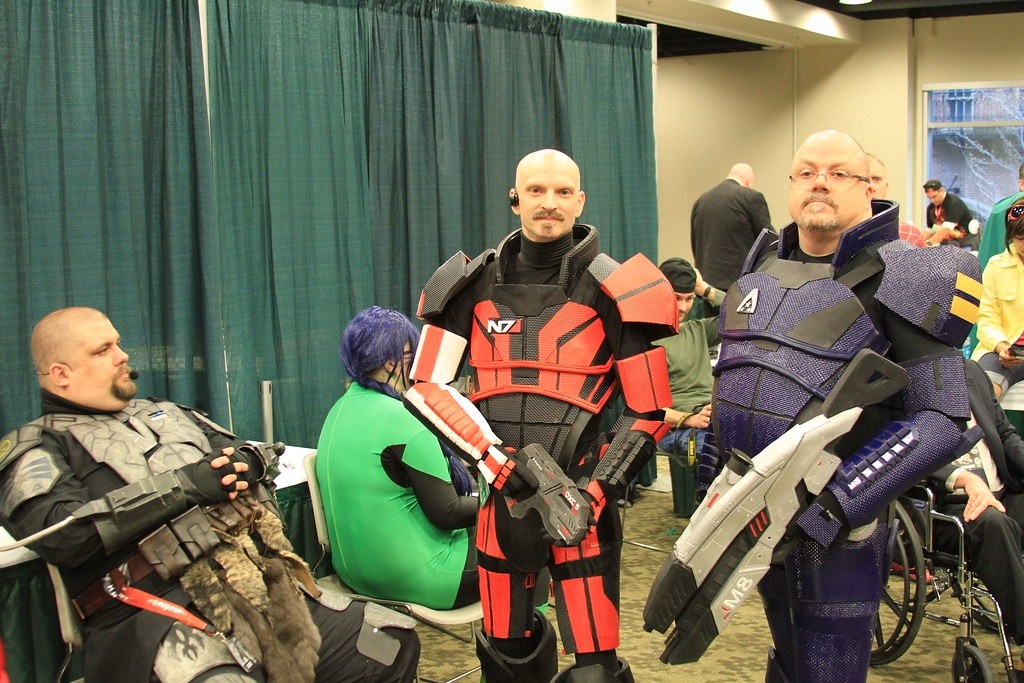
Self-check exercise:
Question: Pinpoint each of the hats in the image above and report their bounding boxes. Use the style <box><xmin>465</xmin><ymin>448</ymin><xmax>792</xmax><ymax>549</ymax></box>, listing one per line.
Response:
<box><xmin>660</xmin><ymin>257</ymin><xmax>697</xmax><ymax>292</ymax></box>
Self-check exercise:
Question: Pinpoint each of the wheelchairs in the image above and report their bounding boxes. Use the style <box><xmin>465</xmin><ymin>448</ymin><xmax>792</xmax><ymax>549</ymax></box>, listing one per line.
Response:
<box><xmin>867</xmin><ymin>483</ymin><xmax>1024</xmax><ymax>683</ymax></box>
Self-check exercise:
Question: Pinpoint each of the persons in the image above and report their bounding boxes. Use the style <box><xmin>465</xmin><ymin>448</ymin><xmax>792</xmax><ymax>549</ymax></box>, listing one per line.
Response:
<box><xmin>403</xmin><ymin>150</ymin><xmax>678</xmax><ymax>683</ymax></box>
<box><xmin>968</xmin><ymin>162</ymin><xmax>1024</xmax><ymax>401</ymax></box>
<box><xmin>923</xmin><ymin>179</ymin><xmax>977</xmax><ymax>251</ymax></box>
<box><xmin>691</xmin><ymin>164</ymin><xmax>776</xmax><ymax>292</ymax></box>
<box><xmin>922</xmin><ymin>360</ymin><xmax>1024</xmax><ymax>670</ymax></box>
<box><xmin>865</xmin><ymin>153</ymin><xmax>926</xmax><ymax>247</ymax></box>
<box><xmin>0</xmin><ymin>307</ymin><xmax>421</xmax><ymax>683</ymax></box>
<box><xmin>651</xmin><ymin>258</ymin><xmax>726</xmax><ymax>461</ymax></box>
<box><xmin>314</xmin><ymin>307</ymin><xmax>550</xmax><ymax>618</ymax></box>
<box><xmin>703</xmin><ymin>129</ymin><xmax>983</xmax><ymax>683</ymax></box>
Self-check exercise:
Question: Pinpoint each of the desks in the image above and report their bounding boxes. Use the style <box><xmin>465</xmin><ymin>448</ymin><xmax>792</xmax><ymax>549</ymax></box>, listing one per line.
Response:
<box><xmin>0</xmin><ymin>430</ymin><xmax>323</xmax><ymax>683</ymax></box>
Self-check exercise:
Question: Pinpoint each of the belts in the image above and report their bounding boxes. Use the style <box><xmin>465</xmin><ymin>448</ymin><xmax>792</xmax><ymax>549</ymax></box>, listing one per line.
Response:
<box><xmin>74</xmin><ymin>553</ymin><xmax>157</xmax><ymax>619</ymax></box>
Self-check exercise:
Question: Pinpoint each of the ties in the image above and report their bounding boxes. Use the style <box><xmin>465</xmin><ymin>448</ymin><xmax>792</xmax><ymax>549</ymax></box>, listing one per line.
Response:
<box><xmin>956</xmin><ymin>428</ymin><xmax>990</xmax><ymax>489</ymax></box>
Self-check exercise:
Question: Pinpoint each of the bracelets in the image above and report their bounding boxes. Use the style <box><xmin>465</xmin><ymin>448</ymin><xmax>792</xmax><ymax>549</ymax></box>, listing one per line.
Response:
<box><xmin>702</xmin><ymin>284</ymin><xmax>713</xmax><ymax>300</ymax></box>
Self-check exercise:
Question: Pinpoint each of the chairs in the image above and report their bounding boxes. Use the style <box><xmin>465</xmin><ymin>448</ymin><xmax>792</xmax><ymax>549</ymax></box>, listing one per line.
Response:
<box><xmin>303</xmin><ymin>448</ymin><xmax>490</xmax><ymax>683</ymax></box>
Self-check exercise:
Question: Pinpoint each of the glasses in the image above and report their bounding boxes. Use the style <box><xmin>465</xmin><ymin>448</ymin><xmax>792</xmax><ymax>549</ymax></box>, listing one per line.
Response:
<box><xmin>1007</xmin><ymin>205</ymin><xmax>1024</xmax><ymax>222</ymax></box>
<box><xmin>923</xmin><ymin>183</ymin><xmax>937</xmax><ymax>189</ymax></box>
<box><xmin>789</xmin><ymin>166</ymin><xmax>870</xmax><ymax>184</ymax></box>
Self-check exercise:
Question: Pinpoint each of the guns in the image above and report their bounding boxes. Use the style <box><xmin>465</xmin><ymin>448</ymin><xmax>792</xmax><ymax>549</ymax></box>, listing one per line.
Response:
<box><xmin>503</xmin><ymin>443</ymin><xmax>591</xmax><ymax>546</ymax></box>
<box><xmin>642</xmin><ymin>349</ymin><xmax>910</xmax><ymax>665</ymax></box>
